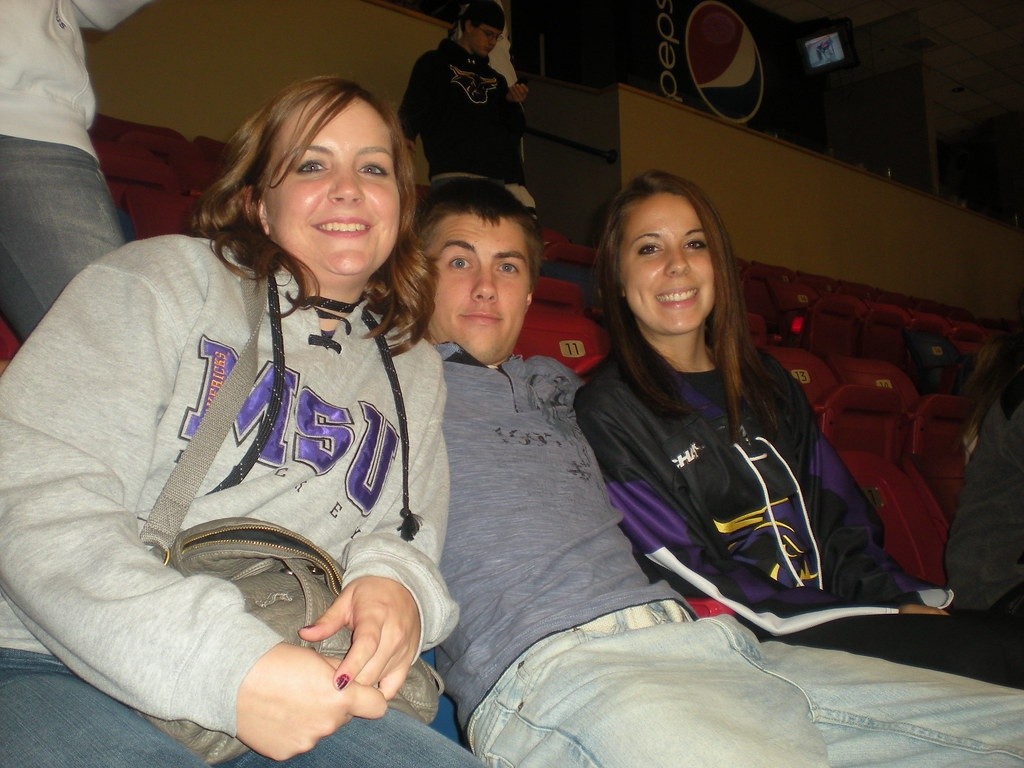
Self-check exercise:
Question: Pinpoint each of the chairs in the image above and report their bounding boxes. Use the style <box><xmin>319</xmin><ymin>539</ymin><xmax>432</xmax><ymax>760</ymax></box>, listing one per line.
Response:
<box><xmin>0</xmin><ymin>114</ymin><xmax>1024</xmax><ymax>616</ymax></box>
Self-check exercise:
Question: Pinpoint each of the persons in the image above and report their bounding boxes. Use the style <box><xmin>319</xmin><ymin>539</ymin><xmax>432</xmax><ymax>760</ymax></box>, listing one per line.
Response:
<box><xmin>397</xmin><ymin>0</ymin><xmax>538</xmax><ymax>221</ymax></box>
<box><xmin>392</xmin><ymin>173</ymin><xmax>1024</xmax><ymax>768</ymax></box>
<box><xmin>942</xmin><ymin>295</ymin><xmax>1024</xmax><ymax>618</ymax></box>
<box><xmin>0</xmin><ymin>0</ymin><xmax>149</xmax><ymax>342</ymax></box>
<box><xmin>0</xmin><ymin>75</ymin><xmax>484</xmax><ymax>768</ymax></box>
<box><xmin>573</xmin><ymin>170</ymin><xmax>1024</xmax><ymax>690</ymax></box>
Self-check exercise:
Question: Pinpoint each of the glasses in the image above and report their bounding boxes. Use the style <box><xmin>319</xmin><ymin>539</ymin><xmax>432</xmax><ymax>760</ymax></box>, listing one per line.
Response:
<box><xmin>478</xmin><ymin>27</ymin><xmax>504</xmax><ymax>42</ymax></box>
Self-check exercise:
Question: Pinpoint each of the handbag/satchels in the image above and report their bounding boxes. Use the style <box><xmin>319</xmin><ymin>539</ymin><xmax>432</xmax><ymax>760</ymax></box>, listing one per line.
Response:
<box><xmin>128</xmin><ymin>516</ymin><xmax>443</xmax><ymax>766</ymax></box>
